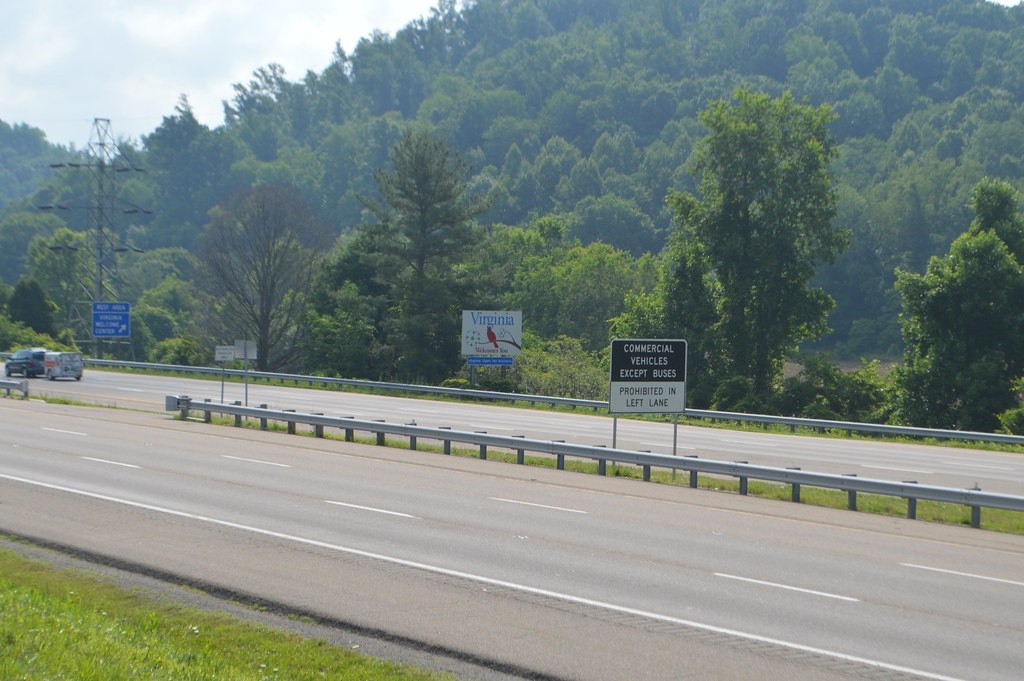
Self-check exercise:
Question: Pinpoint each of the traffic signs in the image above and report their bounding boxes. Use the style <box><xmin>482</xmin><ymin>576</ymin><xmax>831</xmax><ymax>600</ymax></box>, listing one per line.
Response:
<box><xmin>92</xmin><ymin>312</ymin><xmax>132</xmax><ymax>338</ymax></box>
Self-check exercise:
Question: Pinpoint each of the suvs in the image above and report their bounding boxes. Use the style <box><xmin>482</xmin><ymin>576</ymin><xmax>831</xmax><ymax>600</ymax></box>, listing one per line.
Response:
<box><xmin>5</xmin><ymin>347</ymin><xmax>52</xmax><ymax>379</ymax></box>
